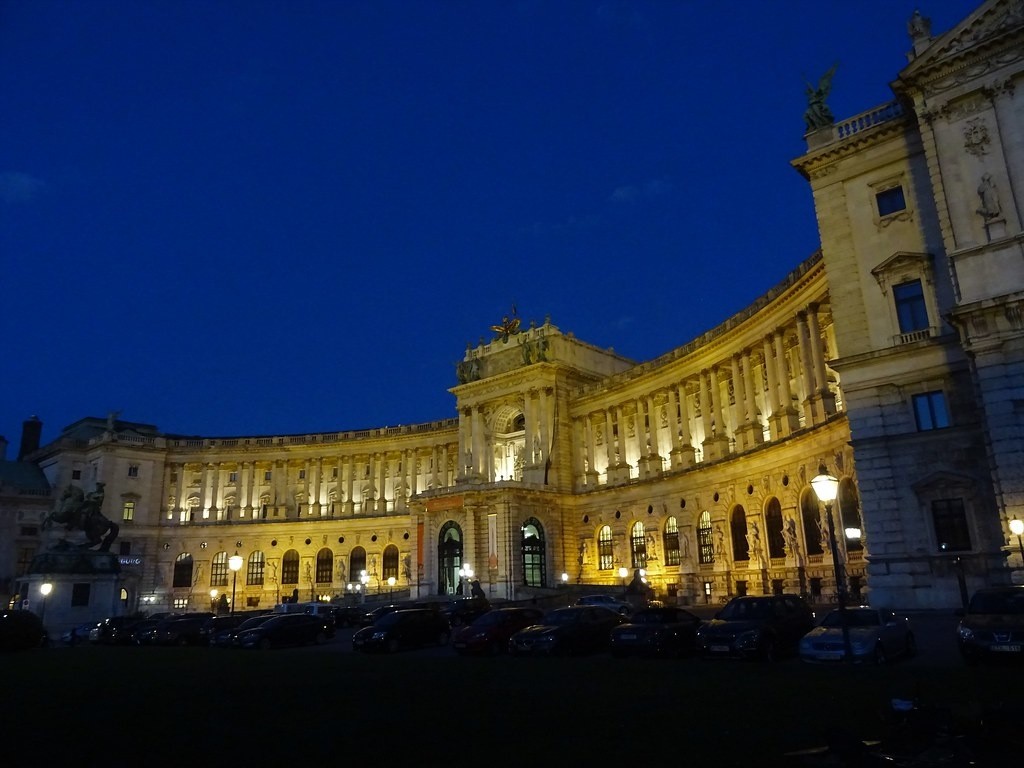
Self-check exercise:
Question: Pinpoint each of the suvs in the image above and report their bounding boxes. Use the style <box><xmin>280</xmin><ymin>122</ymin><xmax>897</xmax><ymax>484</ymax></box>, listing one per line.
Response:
<box><xmin>694</xmin><ymin>592</ymin><xmax>814</xmax><ymax>669</ymax></box>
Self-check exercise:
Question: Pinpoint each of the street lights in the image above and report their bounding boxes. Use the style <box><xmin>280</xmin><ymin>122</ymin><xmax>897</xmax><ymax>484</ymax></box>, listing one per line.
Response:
<box><xmin>1008</xmin><ymin>513</ymin><xmax>1024</xmax><ymax>566</ymax></box>
<box><xmin>228</xmin><ymin>550</ymin><xmax>244</xmax><ymax>615</ymax></box>
<box><xmin>39</xmin><ymin>577</ymin><xmax>54</xmax><ymax>628</ymax></box>
<box><xmin>808</xmin><ymin>457</ymin><xmax>854</xmax><ymax>662</ymax></box>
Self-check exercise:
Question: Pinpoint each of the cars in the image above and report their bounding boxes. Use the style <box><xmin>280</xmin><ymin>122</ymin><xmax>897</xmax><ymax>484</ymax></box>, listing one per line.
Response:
<box><xmin>0</xmin><ymin>596</ymin><xmax>488</xmax><ymax>668</ymax></box>
<box><xmin>574</xmin><ymin>595</ymin><xmax>634</xmax><ymax>622</ymax></box>
<box><xmin>610</xmin><ymin>609</ymin><xmax>701</xmax><ymax>661</ymax></box>
<box><xmin>798</xmin><ymin>604</ymin><xmax>917</xmax><ymax>678</ymax></box>
<box><xmin>508</xmin><ymin>606</ymin><xmax>629</xmax><ymax>672</ymax></box>
<box><xmin>957</xmin><ymin>586</ymin><xmax>1024</xmax><ymax>662</ymax></box>
<box><xmin>451</xmin><ymin>608</ymin><xmax>542</xmax><ymax>659</ymax></box>
